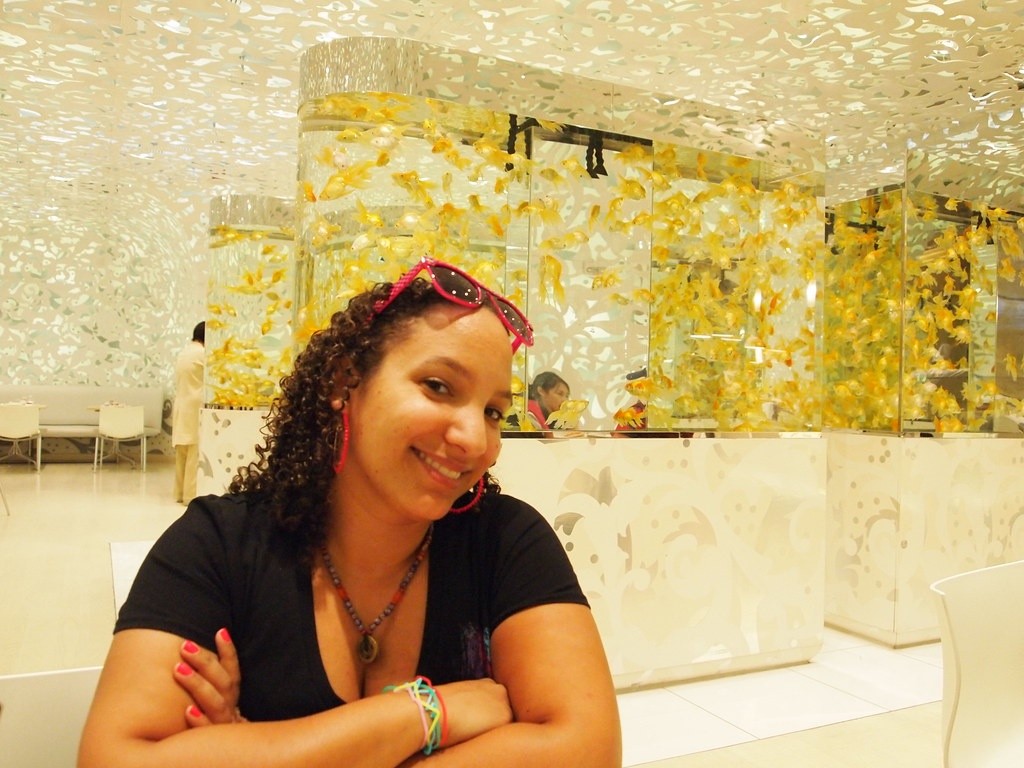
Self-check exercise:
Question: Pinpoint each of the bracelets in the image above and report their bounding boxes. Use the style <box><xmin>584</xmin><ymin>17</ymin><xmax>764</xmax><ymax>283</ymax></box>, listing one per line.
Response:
<box><xmin>384</xmin><ymin>676</ymin><xmax>447</xmax><ymax>756</ymax></box>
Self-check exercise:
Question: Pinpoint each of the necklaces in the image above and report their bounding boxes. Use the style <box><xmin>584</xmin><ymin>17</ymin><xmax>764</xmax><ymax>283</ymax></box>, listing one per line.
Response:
<box><xmin>319</xmin><ymin>521</ymin><xmax>434</xmax><ymax>664</ymax></box>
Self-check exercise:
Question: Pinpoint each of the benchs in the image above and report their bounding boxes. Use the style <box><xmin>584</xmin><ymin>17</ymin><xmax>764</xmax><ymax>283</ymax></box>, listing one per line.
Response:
<box><xmin>0</xmin><ymin>383</ymin><xmax>164</xmax><ymax>468</ymax></box>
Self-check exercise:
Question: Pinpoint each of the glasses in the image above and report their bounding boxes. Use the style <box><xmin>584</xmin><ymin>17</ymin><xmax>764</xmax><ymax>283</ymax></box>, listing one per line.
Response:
<box><xmin>356</xmin><ymin>256</ymin><xmax>534</xmax><ymax>359</ymax></box>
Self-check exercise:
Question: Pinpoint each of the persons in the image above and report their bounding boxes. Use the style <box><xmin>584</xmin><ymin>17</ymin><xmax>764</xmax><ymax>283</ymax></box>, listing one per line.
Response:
<box><xmin>500</xmin><ymin>371</ymin><xmax>570</xmax><ymax>438</ymax></box>
<box><xmin>78</xmin><ymin>254</ymin><xmax>624</xmax><ymax>768</ymax></box>
<box><xmin>612</xmin><ymin>369</ymin><xmax>696</xmax><ymax>437</ymax></box>
<box><xmin>170</xmin><ymin>321</ymin><xmax>205</xmax><ymax>504</ymax></box>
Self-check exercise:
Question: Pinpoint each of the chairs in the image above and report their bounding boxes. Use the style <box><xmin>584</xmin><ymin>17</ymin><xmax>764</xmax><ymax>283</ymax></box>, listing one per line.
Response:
<box><xmin>0</xmin><ymin>404</ymin><xmax>42</xmax><ymax>472</ymax></box>
<box><xmin>931</xmin><ymin>562</ymin><xmax>1024</xmax><ymax>768</ymax></box>
<box><xmin>92</xmin><ymin>404</ymin><xmax>148</xmax><ymax>474</ymax></box>
